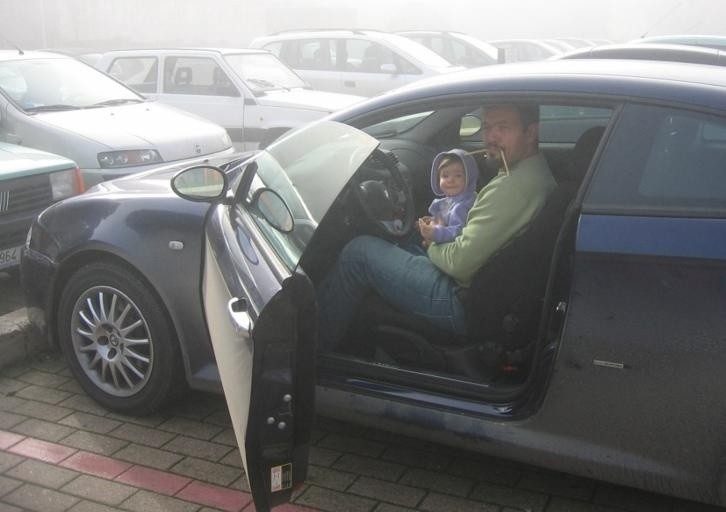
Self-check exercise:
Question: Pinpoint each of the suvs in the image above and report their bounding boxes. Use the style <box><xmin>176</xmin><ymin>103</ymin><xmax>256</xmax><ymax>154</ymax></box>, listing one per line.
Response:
<box><xmin>390</xmin><ymin>30</ymin><xmax>503</xmax><ymax>66</ymax></box>
<box><xmin>0</xmin><ymin>32</ymin><xmax>235</xmax><ymax>189</ymax></box>
<box><xmin>96</xmin><ymin>48</ymin><xmax>372</xmax><ymax>152</ymax></box>
<box><xmin>248</xmin><ymin>29</ymin><xmax>467</xmax><ymax>97</ymax></box>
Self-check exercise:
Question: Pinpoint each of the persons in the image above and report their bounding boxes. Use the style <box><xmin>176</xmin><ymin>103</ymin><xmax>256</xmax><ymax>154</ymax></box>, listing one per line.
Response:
<box><xmin>317</xmin><ymin>101</ymin><xmax>557</xmax><ymax>355</ymax></box>
<box><xmin>415</xmin><ymin>149</ymin><xmax>479</xmax><ymax>250</ymax></box>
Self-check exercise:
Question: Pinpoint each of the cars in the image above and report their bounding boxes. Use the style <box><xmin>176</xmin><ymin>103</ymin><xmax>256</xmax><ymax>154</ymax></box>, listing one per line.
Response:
<box><xmin>20</xmin><ymin>60</ymin><xmax>726</xmax><ymax>512</ymax></box>
